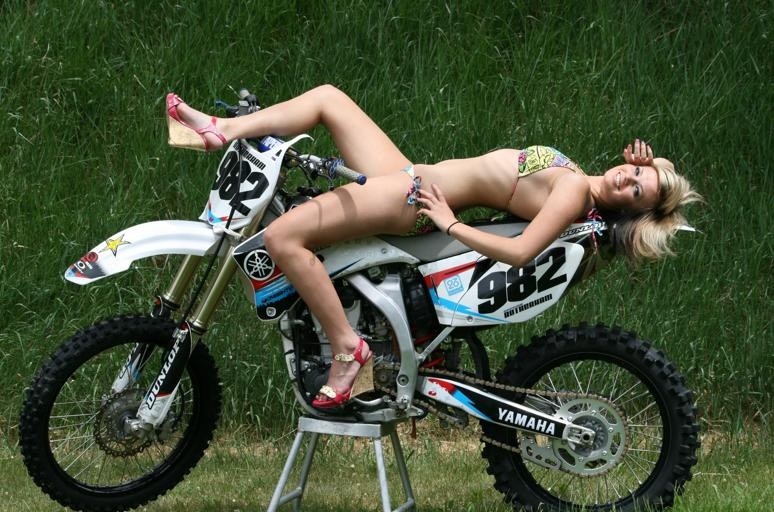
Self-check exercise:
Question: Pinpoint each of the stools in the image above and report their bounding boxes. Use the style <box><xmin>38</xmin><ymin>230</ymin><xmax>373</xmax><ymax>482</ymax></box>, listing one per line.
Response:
<box><xmin>265</xmin><ymin>412</ymin><xmax>420</xmax><ymax>512</ymax></box>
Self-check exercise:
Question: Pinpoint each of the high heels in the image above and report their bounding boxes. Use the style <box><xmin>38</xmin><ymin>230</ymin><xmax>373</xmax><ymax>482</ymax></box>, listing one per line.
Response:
<box><xmin>165</xmin><ymin>92</ymin><xmax>228</xmax><ymax>153</ymax></box>
<box><xmin>312</xmin><ymin>337</ymin><xmax>376</xmax><ymax>410</ymax></box>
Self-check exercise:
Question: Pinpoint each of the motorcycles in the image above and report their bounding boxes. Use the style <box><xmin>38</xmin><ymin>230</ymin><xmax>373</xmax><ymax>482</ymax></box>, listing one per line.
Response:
<box><xmin>20</xmin><ymin>82</ymin><xmax>703</xmax><ymax>512</ymax></box>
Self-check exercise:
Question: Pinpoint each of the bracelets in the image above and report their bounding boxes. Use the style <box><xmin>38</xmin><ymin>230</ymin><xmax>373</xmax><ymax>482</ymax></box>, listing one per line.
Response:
<box><xmin>447</xmin><ymin>221</ymin><xmax>460</xmax><ymax>235</ymax></box>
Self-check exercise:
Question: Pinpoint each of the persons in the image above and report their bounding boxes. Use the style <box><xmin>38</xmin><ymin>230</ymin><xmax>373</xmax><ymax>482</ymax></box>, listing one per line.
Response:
<box><xmin>165</xmin><ymin>83</ymin><xmax>707</xmax><ymax>408</ymax></box>
